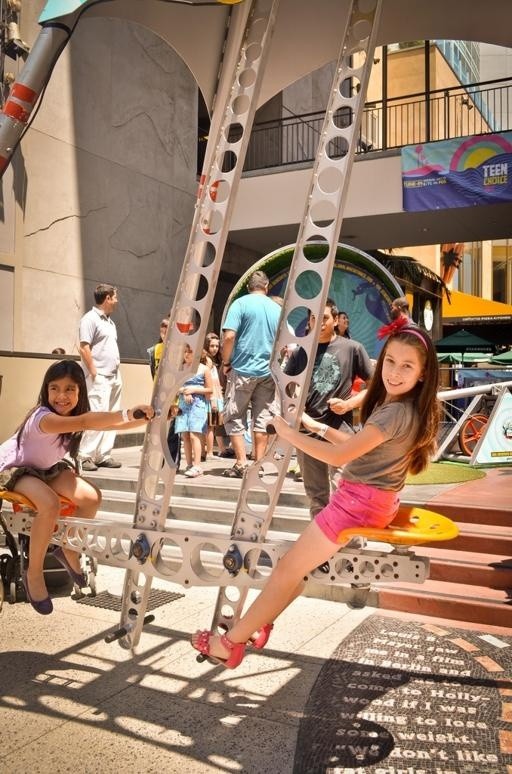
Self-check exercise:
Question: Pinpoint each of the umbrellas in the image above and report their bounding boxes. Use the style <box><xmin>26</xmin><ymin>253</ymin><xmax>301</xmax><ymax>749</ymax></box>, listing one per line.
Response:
<box><xmin>491</xmin><ymin>351</ymin><xmax>512</xmax><ymax>365</ymax></box>
<box><xmin>433</xmin><ymin>329</ymin><xmax>496</xmax><ymax>368</ymax></box>
<box><xmin>436</xmin><ymin>353</ymin><xmax>493</xmax><ymax>364</ymax></box>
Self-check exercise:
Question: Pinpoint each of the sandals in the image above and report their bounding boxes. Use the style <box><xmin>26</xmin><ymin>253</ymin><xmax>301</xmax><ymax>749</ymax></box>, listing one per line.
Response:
<box><xmin>190</xmin><ymin>631</ymin><xmax>247</xmax><ymax>670</ymax></box>
<box><xmin>219</xmin><ymin>615</ymin><xmax>273</xmax><ymax>649</ymax></box>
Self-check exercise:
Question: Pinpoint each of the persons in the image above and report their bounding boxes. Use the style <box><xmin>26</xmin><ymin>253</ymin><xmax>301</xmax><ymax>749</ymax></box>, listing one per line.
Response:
<box><xmin>220</xmin><ymin>271</ymin><xmax>282</xmax><ymax>478</ymax></box>
<box><xmin>190</xmin><ymin>318</ymin><xmax>441</xmax><ymax>670</ymax></box>
<box><xmin>284</xmin><ymin>298</ymin><xmax>373</xmax><ymax>548</ymax></box>
<box><xmin>78</xmin><ymin>283</ymin><xmax>122</xmax><ymax>471</ymax></box>
<box><xmin>334</xmin><ymin>312</ymin><xmax>350</xmax><ymax>339</ymax></box>
<box><xmin>247</xmin><ymin>295</ymin><xmax>283</xmax><ymax>460</ymax></box>
<box><xmin>146</xmin><ymin>318</ymin><xmax>234</xmax><ymax>479</ymax></box>
<box><xmin>391</xmin><ymin>297</ymin><xmax>415</xmax><ymax>325</ymax></box>
<box><xmin>0</xmin><ymin>358</ymin><xmax>179</xmax><ymax>615</ymax></box>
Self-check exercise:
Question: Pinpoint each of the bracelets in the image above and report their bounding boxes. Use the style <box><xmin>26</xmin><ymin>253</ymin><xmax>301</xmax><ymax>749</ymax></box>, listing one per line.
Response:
<box><xmin>223</xmin><ymin>362</ymin><xmax>230</xmax><ymax>366</ymax></box>
<box><xmin>122</xmin><ymin>408</ymin><xmax>131</xmax><ymax>423</ymax></box>
<box><xmin>318</xmin><ymin>424</ymin><xmax>329</xmax><ymax>437</ymax></box>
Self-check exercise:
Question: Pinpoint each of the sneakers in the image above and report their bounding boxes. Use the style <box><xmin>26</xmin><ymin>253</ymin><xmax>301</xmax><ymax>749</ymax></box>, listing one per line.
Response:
<box><xmin>81</xmin><ymin>447</ymin><xmax>304</xmax><ymax>482</ymax></box>
<box><xmin>21</xmin><ymin>570</ymin><xmax>53</xmax><ymax>615</ymax></box>
<box><xmin>51</xmin><ymin>546</ymin><xmax>88</xmax><ymax>588</ymax></box>
